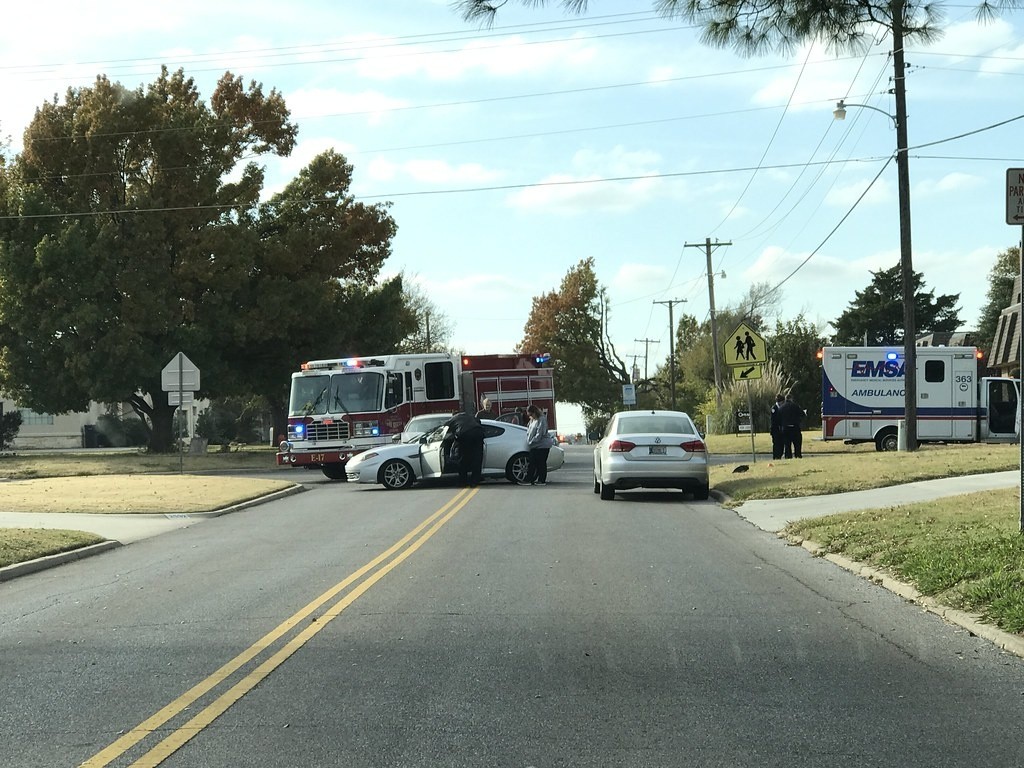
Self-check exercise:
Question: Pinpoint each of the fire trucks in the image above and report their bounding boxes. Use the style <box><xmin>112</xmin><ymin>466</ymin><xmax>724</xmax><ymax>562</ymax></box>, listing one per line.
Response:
<box><xmin>276</xmin><ymin>352</ymin><xmax>559</xmax><ymax>481</ymax></box>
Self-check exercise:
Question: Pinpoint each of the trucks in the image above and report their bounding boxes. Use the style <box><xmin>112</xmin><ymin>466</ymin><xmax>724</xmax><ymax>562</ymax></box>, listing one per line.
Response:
<box><xmin>821</xmin><ymin>346</ymin><xmax>1021</xmax><ymax>452</ymax></box>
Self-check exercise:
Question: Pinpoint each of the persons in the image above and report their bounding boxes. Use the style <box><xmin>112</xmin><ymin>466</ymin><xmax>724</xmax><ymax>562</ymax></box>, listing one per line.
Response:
<box><xmin>770</xmin><ymin>394</ymin><xmax>786</xmax><ymax>461</ymax></box>
<box><xmin>517</xmin><ymin>404</ymin><xmax>553</xmax><ymax>487</ymax></box>
<box><xmin>777</xmin><ymin>395</ymin><xmax>807</xmax><ymax>459</ymax></box>
<box><xmin>442</xmin><ymin>411</ymin><xmax>486</xmax><ymax>490</ymax></box>
<box><xmin>475</xmin><ymin>398</ymin><xmax>497</xmax><ymax>420</ymax></box>
<box><xmin>511</xmin><ymin>406</ymin><xmax>531</xmax><ymax>428</ymax></box>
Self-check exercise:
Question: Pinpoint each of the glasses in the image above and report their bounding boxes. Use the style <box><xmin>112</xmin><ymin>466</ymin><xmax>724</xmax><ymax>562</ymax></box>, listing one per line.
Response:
<box><xmin>529</xmin><ymin>413</ymin><xmax>534</xmax><ymax>416</ymax></box>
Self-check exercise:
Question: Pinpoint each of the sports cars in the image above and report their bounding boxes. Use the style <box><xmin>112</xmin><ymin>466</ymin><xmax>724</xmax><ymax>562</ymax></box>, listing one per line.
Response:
<box><xmin>345</xmin><ymin>418</ymin><xmax>565</xmax><ymax>490</ymax></box>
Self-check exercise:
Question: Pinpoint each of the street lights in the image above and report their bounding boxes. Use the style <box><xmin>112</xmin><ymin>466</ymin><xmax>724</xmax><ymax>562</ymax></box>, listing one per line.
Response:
<box><xmin>831</xmin><ymin>99</ymin><xmax>917</xmax><ymax>452</ymax></box>
<box><xmin>704</xmin><ymin>269</ymin><xmax>727</xmax><ymax>409</ymax></box>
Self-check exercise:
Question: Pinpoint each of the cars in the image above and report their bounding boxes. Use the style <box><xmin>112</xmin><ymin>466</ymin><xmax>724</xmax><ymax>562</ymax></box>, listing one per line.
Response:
<box><xmin>391</xmin><ymin>413</ymin><xmax>454</xmax><ymax>445</ymax></box>
<box><xmin>588</xmin><ymin>407</ymin><xmax>710</xmax><ymax>500</ymax></box>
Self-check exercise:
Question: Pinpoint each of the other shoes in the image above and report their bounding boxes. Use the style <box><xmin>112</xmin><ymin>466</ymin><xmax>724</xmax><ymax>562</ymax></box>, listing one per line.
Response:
<box><xmin>470</xmin><ymin>481</ymin><xmax>477</xmax><ymax>488</ymax></box>
<box><xmin>455</xmin><ymin>482</ymin><xmax>466</xmax><ymax>487</ymax></box>
<box><xmin>535</xmin><ymin>482</ymin><xmax>546</xmax><ymax>485</ymax></box>
<box><xmin>517</xmin><ymin>481</ymin><xmax>532</xmax><ymax>485</ymax></box>
